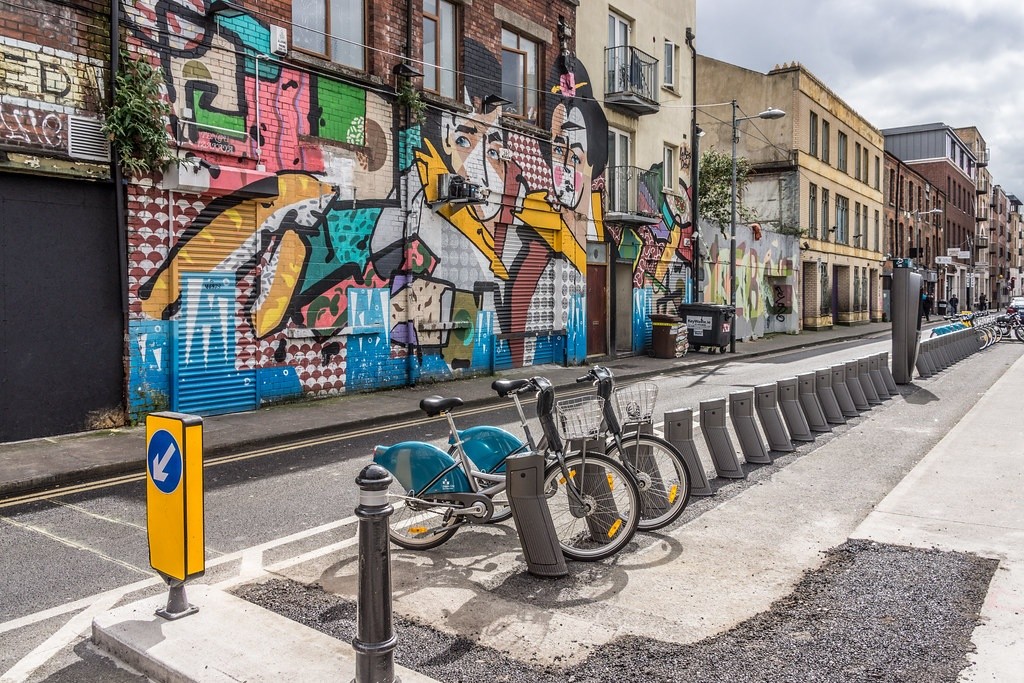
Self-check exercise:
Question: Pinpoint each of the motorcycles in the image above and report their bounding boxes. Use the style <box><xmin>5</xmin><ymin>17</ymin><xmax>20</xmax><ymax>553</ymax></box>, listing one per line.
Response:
<box><xmin>994</xmin><ymin>310</ymin><xmax>1024</xmax><ymax>343</ymax></box>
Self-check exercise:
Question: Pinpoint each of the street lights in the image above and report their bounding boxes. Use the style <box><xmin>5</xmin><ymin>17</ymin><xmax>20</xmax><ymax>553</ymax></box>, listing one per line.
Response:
<box><xmin>916</xmin><ymin>207</ymin><xmax>943</xmax><ymax>276</ymax></box>
<box><xmin>728</xmin><ymin>99</ymin><xmax>786</xmax><ymax>352</ymax></box>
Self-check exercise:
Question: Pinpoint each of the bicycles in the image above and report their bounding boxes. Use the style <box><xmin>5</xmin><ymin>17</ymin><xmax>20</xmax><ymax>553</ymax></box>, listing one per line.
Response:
<box><xmin>445</xmin><ymin>365</ymin><xmax>692</xmax><ymax>531</ymax></box>
<box><xmin>368</xmin><ymin>375</ymin><xmax>642</xmax><ymax>561</ymax></box>
<box><xmin>929</xmin><ymin>310</ymin><xmax>1003</xmax><ymax>350</ymax></box>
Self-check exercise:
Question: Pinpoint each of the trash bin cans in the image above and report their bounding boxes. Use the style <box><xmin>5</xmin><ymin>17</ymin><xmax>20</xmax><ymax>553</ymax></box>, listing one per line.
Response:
<box><xmin>680</xmin><ymin>302</ymin><xmax>736</xmax><ymax>355</ymax></box>
<box><xmin>648</xmin><ymin>314</ymin><xmax>683</xmax><ymax>358</ymax></box>
<box><xmin>938</xmin><ymin>300</ymin><xmax>948</xmax><ymax>316</ymax></box>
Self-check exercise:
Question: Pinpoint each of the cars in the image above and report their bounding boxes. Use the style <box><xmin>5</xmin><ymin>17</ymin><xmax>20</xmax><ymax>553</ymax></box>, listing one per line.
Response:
<box><xmin>1005</xmin><ymin>296</ymin><xmax>1024</xmax><ymax>323</ymax></box>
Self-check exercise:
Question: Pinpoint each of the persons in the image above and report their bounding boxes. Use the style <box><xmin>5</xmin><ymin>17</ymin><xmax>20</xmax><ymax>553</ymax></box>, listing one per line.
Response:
<box><xmin>949</xmin><ymin>294</ymin><xmax>958</xmax><ymax>316</ymax></box>
<box><xmin>979</xmin><ymin>293</ymin><xmax>985</xmax><ymax>311</ymax></box>
<box><xmin>925</xmin><ymin>294</ymin><xmax>931</xmax><ymax>301</ymax></box>
<box><xmin>922</xmin><ymin>296</ymin><xmax>933</xmax><ymax>322</ymax></box>
<box><xmin>983</xmin><ymin>295</ymin><xmax>988</xmax><ymax>311</ymax></box>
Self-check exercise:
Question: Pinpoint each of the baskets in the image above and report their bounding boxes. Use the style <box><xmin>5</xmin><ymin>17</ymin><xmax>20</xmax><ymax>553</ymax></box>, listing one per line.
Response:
<box><xmin>557</xmin><ymin>395</ymin><xmax>605</xmax><ymax>441</ymax></box>
<box><xmin>613</xmin><ymin>381</ymin><xmax>658</xmax><ymax>426</ymax></box>
<box><xmin>975</xmin><ymin>314</ymin><xmax>996</xmax><ymax>326</ymax></box>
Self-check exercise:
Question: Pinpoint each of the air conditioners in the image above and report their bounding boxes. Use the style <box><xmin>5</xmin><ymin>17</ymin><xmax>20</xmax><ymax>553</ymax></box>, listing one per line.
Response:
<box><xmin>436</xmin><ymin>172</ymin><xmax>459</xmax><ymax>200</ymax></box>
<box><xmin>561</xmin><ymin>26</ymin><xmax>571</xmax><ymax>37</ymax></box>
